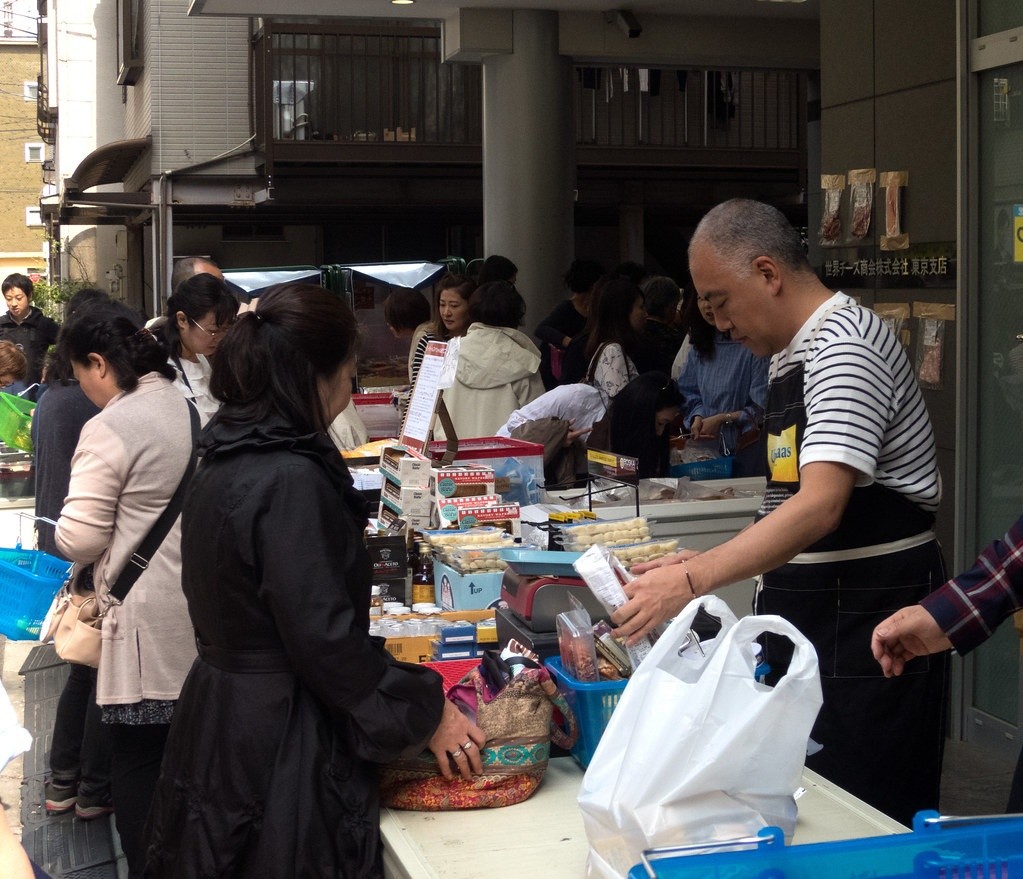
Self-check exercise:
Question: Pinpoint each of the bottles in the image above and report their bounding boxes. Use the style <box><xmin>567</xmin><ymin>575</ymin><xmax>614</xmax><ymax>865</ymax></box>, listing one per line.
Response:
<box><xmin>371</xmin><ymin>536</ymin><xmax>444</xmax><ymax>638</ymax></box>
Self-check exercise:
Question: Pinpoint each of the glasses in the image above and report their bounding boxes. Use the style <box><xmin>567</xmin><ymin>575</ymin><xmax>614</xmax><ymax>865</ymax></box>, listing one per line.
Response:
<box><xmin>192</xmin><ymin>318</ymin><xmax>229</xmax><ymax>338</ymax></box>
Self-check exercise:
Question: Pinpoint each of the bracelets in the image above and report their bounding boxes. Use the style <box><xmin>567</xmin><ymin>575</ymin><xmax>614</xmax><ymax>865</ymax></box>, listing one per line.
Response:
<box><xmin>726</xmin><ymin>413</ymin><xmax>732</xmax><ymax>424</ymax></box>
<box><xmin>681</xmin><ymin>559</ymin><xmax>697</xmax><ymax>599</ymax></box>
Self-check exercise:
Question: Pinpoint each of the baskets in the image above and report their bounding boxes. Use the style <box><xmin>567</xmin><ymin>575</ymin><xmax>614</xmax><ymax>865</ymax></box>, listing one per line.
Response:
<box><xmin>668</xmin><ymin>431</ymin><xmax>737</xmax><ymax>481</ymax></box>
<box><xmin>0</xmin><ymin>511</ymin><xmax>73</xmax><ymax>641</ymax></box>
<box><xmin>543</xmin><ymin>655</ymin><xmax>773</xmax><ymax>774</ymax></box>
<box><xmin>549</xmin><ymin>340</ymin><xmax>568</xmax><ymax>380</ymax></box>
<box><xmin>0</xmin><ymin>383</ymin><xmax>40</xmax><ymax>453</ymax></box>
<box><xmin>628</xmin><ymin>810</ymin><xmax>1023</xmax><ymax>879</ymax></box>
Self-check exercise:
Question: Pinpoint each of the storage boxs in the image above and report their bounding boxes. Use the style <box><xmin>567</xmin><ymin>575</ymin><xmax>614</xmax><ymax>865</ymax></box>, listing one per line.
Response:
<box><xmin>379</xmin><ymin>510</ymin><xmax>431</xmax><ymax>532</ymax></box>
<box><xmin>383</xmin><ymin>481</ymin><xmax>431</xmax><ymax>518</ymax></box>
<box><xmin>428</xmin><ymin>556</ymin><xmax>504</xmax><ymax>615</ymax></box>
<box><xmin>376</xmin><ymin>443</ymin><xmax>431</xmax><ymax>489</ymax></box>
<box><xmin>429</xmin><ymin>435</ymin><xmax>545</xmax><ymax>505</ymax></box>
<box><xmin>350</xmin><ymin>393</ymin><xmax>399</xmax><ymax>442</ymax></box>
<box><xmin>415</xmin><ymin>659</ymin><xmax>497</xmax><ymax>715</ymax></box>
<box><xmin>432</xmin><ymin>465</ymin><xmax>521</xmax><ymax>539</ymax></box>
<box><xmin>374</xmin><ymin>609</ymin><xmax>499</xmax><ymax>663</ymax></box>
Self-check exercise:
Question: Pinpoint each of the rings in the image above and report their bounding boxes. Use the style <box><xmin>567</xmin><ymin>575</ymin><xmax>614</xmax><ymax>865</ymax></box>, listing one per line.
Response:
<box><xmin>452</xmin><ymin>749</ymin><xmax>462</xmax><ymax>757</ymax></box>
<box><xmin>464</xmin><ymin>742</ymin><xmax>472</xmax><ymax>750</ymax></box>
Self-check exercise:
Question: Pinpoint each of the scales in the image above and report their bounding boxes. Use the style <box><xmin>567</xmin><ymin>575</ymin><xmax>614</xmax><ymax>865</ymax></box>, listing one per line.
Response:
<box><xmin>498</xmin><ymin>547</ymin><xmax>616</xmax><ymax>631</ymax></box>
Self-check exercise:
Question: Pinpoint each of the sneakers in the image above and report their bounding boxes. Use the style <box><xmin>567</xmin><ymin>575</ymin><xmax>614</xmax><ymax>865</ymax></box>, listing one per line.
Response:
<box><xmin>75</xmin><ymin>780</ymin><xmax>115</xmax><ymax>820</ymax></box>
<box><xmin>44</xmin><ymin>776</ymin><xmax>78</xmax><ymax>816</ymax></box>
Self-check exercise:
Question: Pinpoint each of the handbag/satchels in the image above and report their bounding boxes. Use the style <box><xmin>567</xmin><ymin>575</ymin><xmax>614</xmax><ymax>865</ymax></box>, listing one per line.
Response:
<box><xmin>577</xmin><ymin>595</ymin><xmax>824</xmax><ymax>879</ymax></box>
<box><xmin>733</xmin><ymin>404</ymin><xmax>769</xmax><ymax>479</ymax></box>
<box><xmin>39</xmin><ymin>562</ymin><xmax>102</xmax><ymax>669</ymax></box>
<box><xmin>379</xmin><ymin>649</ymin><xmax>579</xmax><ymax>812</ymax></box>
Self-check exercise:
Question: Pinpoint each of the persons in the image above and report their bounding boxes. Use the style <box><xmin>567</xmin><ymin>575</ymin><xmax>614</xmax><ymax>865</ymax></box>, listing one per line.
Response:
<box><xmin>870</xmin><ymin>516</ymin><xmax>1023</xmax><ymax>814</ymax></box>
<box><xmin>609</xmin><ymin>197</ymin><xmax>953</xmax><ymax>832</ymax></box>
<box><xmin>381</xmin><ymin>255</ymin><xmax>773</xmax><ymax>486</ymax></box>
<box><xmin>138</xmin><ymin>280</ymin><xmax>486</xmax><ymax>879</ymax></box>
<box><xmin>54</xmin><ymin>311</ymin><xmax>212</xmax><ymax>879</ymax></box>
<box><xmin>1</xmin><ymin>257</ymin><xmax>368</xmax><ymax>821</ymax></box>
